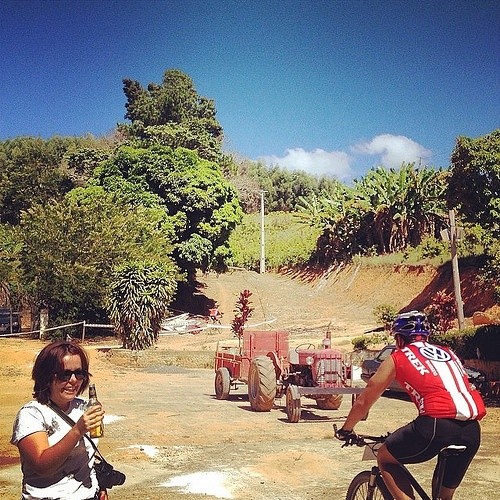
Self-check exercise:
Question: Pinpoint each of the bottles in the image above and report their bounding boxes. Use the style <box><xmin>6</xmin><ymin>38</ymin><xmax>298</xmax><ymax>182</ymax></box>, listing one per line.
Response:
<box><xmin>87</xmin><ymin>384</ymin><xmax>102</xmax><ymax>438</ymax></box>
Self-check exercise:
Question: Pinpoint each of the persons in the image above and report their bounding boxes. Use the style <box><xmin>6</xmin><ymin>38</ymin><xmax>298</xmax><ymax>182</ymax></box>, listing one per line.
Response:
<box><xmin>336</xmin><ymin>311</ymin><xmax>487</xmax><ymax>500</ymax></box>
<box><xmin>10</xmin><ymin>340</ymin><xmax>108</xmax><ymax>500</ymax></box>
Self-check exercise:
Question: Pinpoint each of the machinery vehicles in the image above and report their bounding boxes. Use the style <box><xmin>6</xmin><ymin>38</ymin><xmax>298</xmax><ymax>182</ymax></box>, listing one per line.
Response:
<box><xmin>213</xmin><ymin>329</ymin><xmax>370</xmax><ymax>422</ymax></box>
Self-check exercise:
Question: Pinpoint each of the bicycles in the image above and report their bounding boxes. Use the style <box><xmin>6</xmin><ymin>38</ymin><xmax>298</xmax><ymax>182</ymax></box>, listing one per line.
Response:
<box><xmin>333</xmin><ymin>423</ymin><xmax>467</xmax><ymax>500</ymax></box>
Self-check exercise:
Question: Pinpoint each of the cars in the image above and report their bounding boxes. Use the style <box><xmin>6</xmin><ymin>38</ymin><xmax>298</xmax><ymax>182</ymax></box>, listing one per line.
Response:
<box><xmin>361</xmin><ymin>345</ymin><xmax>486</xmax><ymax>402</ymax></box>
<box><xmin>0</xmin><ymin>308</ymin><xmax>24</xmax><ymax>334</ymax></box>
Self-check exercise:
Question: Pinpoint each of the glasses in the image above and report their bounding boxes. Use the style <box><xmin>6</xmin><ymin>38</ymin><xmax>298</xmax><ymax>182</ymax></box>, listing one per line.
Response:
<box><xmin>54</xmin><ymin>371</ymin><xmax>84</xmax><ymax>382</ymax></box>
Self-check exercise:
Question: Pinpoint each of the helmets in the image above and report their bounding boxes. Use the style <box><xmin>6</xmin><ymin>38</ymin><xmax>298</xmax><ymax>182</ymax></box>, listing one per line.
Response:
<box><xmin>389</xmin><ymin>311</ymin><xmax>431</xmax><ymax>337</ymax></box>
